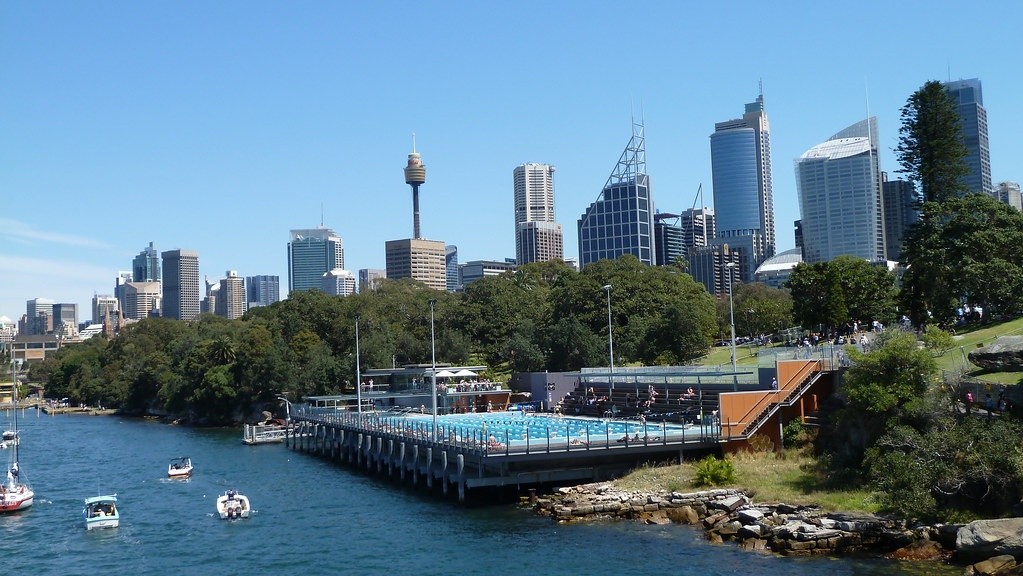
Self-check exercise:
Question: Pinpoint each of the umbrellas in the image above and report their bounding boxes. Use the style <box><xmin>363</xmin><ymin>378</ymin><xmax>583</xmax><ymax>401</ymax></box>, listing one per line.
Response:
<box><xmin>436</xmin><ymin>370</ymin><xmax>479</xmax><ymax>381</ymax></box>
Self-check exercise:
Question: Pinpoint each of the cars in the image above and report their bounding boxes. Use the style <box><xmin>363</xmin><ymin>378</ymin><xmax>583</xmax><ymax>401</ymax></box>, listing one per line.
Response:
<box><xmin>714</xmin><ymin>338</ymin><xmax>748</xmax><ymax>348</ymax></box>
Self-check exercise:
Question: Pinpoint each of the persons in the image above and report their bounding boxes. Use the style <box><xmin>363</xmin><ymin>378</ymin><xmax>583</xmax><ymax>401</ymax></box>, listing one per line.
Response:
<box><xmin>786</xmin><ymin>316</ymin><xmax>882</xmax><ymax>360</ymax></box>
<box><xmin>554</xmin><ymin>397</ymin><xmax>566</xmax><ymax>416</ymax></box>
<box><xmin>769</xmin><ymin>376</ymin><xmax>778</xmax><ymax>391</ymax></box>
<box><xmin>611</xmin><ymin>403</ymin><xmax>618</xmax><ymax>421</ymax></box>
<box><xmin>228</xmin><ymin>490</ymin><xmax>236</xmax><ymax>501</ymax></box>
<box><xmin>96</xmin><ymin>502</ymin><xmax>109</xmax><ymax>514</ymax></box>
<box><xmin>437</xmin><ymin>427</ymin><xmax>501</xmax><ymax>449</ymax></box>
<box><xmin>420</xmin><ymin>402</ymin><xmax>427</xmax><ymax>415</ymax></box>
<box><xmin>438</xmin><ymin>378</ymin><xmax>501</xmax><ymax>393</ymax></box>
<box><xmin>644</xmin><ymin>384</ymin><xmax>719</xmax><ymax>425</ymax></box>
<box><xmin>319</xmin><ymin>415</ymin><xmax>356</xmax><ymax>428</ymax></box>
<box><xmin>617</xmin><ymin>434</ymin><xmax>660</xmax><ymax>445</ymax></box>
<box><xmin>369</xmin><ymin>378</ymin><xmax>374</xmax><ymax>392</ymax></box>
<box><xmin>179</xmin><ymin>459</ymin><xmax>187</xmax><ymax>468</ymax></box>
<box><xmin>729</xmin><ymin>334</ymin><xmax>772</xmax><ymax>348</ymax></box>
<box><xmin>411</xmin><ymin>375</ymin><xmax>426</xmax><ymax>393</ymax></box>
<box><xmin>965</xmin><ymin>387</ymin><xmax>1007</xmax><ymax>419</ymax></box>
<box><xmin>361</xmin><ymin>381</ymin><xmax>366</xmax><ymax>392</ymax></box>
<box><xmin>571</xmin><ymin>437</ymin><xmax>592</xmax><ymax>445</ymax></box>
<box><xmin>586</xmin><ymin>386</ymin><xmax>608</xmax><ymax>417</ymax></box>
<box><xmin>362</xmin><ymin>417</ymin><xmax>428</xmax><ymax>442</ymax></box>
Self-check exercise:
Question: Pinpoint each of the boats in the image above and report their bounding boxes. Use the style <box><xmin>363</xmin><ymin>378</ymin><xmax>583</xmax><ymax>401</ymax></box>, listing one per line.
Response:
<box><xmin>217</xmin><ymin>490</ymin><xmax>250</xmax><ymax>519</ymax></box>
<box><xmin>0</xmin><ymin>430</ymin><xmax>21</xmax><ymax>448</ymax></box>
<box><xmin>168</xmin><ymin>456</ymin><xmax>193</xmax><ymax>479</ymax></box>
<box><xmin>82</xmin><ymin>493</ymin><xmax>120</xmax><ymax>530</ymax></box>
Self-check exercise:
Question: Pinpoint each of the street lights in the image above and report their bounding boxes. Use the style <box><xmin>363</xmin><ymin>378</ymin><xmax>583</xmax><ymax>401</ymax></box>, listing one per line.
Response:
<box><xmin>604</xmin><ymin>285</ymin><xmax>614</xmax><ymax>388</ymax></box>
<box><xmin>727</xmin><ymin>263</ymin><xmax>736</xmax><ymax>373</ymax></box>
<box><xmin>354</xmin><ymin>315</ymin><xmax>362</xmax><ymax>413</ymax></box>
<box><xmin>427</xmin><ymin>298</ymin><xmax>438</xmax><ymax>441</ymax></box>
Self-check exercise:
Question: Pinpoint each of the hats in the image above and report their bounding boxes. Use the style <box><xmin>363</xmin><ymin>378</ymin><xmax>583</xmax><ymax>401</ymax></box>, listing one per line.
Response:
<box><xmin>773</xmin><ymin>378</ymin><xmax>775</xmax><ymax>380</ymax></box>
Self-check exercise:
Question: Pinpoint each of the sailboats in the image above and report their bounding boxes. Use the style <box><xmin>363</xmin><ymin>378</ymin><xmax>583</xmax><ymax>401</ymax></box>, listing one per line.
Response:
<box><xmin>0</xmin><ymin>346</ymin><xmax>34</xmax><ymax>512</ymax></box>
